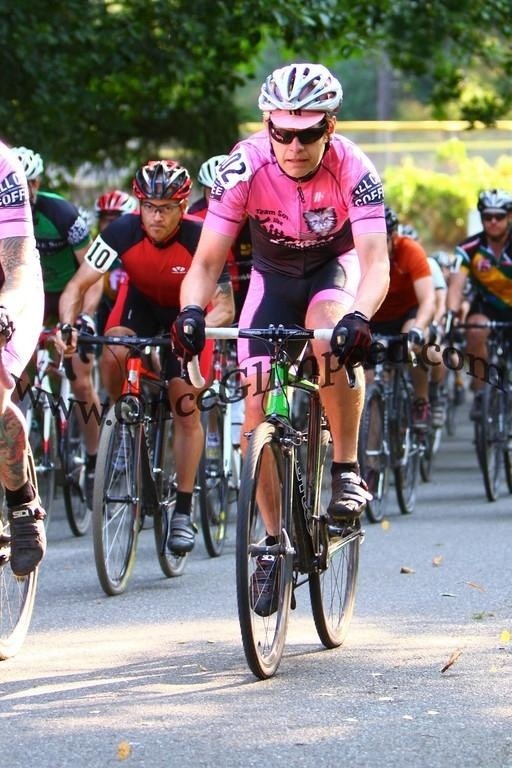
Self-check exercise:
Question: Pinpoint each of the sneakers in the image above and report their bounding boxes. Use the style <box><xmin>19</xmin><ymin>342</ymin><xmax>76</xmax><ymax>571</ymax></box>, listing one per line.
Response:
<box><xmin>5</xmin><ymin>482</ymin><xmax>47</xmax><ymax>579</ymax></box>
<box><xmin>327</xmin><ymin>472</ymin><xmax>374</xmax><ymax>520</ymax></box>
<box><xmin>168</xmin><ymin>510</ymin><xmax>199</xmax><ymax>555</ymax></box>
<box><xmin>248</xmin><ymin>545</ymin><xmax>281</xmax><ymax>619</ymax></box>
<box><xmin>408</xmin><ymin>380</ymin><xmax>484</xmax><ymax>432</ymax></box>
<box><xmin>81</xmin><ymin>468</ymin><xmax>96</xmax><ymax>512</ymax></box>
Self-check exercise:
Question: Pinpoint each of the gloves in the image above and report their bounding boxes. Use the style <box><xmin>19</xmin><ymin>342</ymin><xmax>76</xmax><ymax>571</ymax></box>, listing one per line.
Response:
<box><xmin>169</xmin><ymin>304</ymin><xmax>206</xmax><ymax>367</ymax></box>
<box><xmin>329</xmin><ymin>310</ymin><xmax>376</xmax><ymax>369</ymax></box>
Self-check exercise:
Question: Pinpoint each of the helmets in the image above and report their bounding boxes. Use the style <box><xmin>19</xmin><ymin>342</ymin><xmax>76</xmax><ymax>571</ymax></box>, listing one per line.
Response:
<box><xmin>384</xmin><ymin>204</ymin><xmax>452</xmax><ymax>269</ymax></box>
<box><xmin>258</xmin><ymin>62</ymin><xmax>344</xmax><ymax>130</ymax></box>
<box><xmin>94</xmin><ymin>190</ymin><xmax>139</xmax><ymax>218</ymax></box>
<box><xmin>197</xmin><ymin>154</ymin><xmax>232</xmax><ymax>190</ymax></box>
<box><xmin>9</xmin><ymin>144</ymin><xmax>45</xmax><ymax>182</ymax></box>
<box><xmin>132</xmin><ymin>158</ymin><xmax>194</xmax><ymax>201</ymax></box>
<box><xmin>477</xmin><ymin>188</ymin><xmax>512</xmax><ymax>216</ymax></box>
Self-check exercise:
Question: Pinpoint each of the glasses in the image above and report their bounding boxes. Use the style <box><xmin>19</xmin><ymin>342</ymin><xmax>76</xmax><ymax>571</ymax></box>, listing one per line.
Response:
<box><xmin>482</xmin><ymin>212</ymin><xmax>509</xmax><ymax>221</ymax></box>
<box><xmin>267</xmin><ymin>120</ymin><xmax>328</xmax><ymax>146</ymax></box>
<box><xmin>141</xmin><ymin>201</ymin><xmax>182</xmax><ymax>217</ymax></box>
